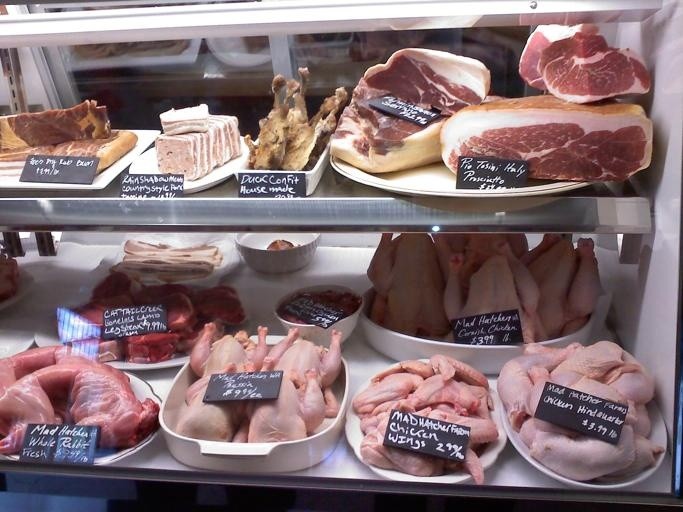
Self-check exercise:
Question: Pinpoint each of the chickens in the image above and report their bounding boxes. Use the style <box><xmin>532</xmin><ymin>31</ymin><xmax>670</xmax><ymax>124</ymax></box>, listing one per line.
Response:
<box><xmin>442</xmin><ymin>239</ymin><xmax>541</xmax><ymax>344</ymax></box>
<box><xmin>520</xmin><ymin>233</ymin><xmax>601</xmax><ymax>341</ymax></box>
<box><xmin>175</xmin><ymin>360</ymin><xmax>257</xmax><ymax>443</ymax></box>
<box><xmin>436</xmin><ymin>233</ymin><xmax>529</xmax><ymax>305</ymax></box>
<box><xmin>245</xmin><ymin>356</ymin><xmax>326</xmax><ymax>443</ymax></box>
<box><xmin>366</xmin><ymin>233</ymin><xmax>454</xmax><ymax>340</ymax></box>
<box><xmin>262</xmin><ymin>327</ymin><xmax>343</xmax><ymax>418</ymax></box>
<box><xmin>189</xmin><ymin>322</ymin><xmax>269</xmax><ymax>378</ymax></box>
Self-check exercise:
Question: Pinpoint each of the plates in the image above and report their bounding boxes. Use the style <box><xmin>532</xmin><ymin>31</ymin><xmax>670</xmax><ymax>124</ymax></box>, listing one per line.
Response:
<box><xmin>129</xmin><ymin>137</ymin><xmax>252</xmax><ymax>193</ymax></box>
<box><xmin>331</xmin><ymin>154</ymin><xmax>597</xmax><ymax>216</ymax></box>
<box><xmin>68</xmin><ymin>38</ymin><xmax>200</xmax><ymax>72</ymax></box>
<box><xmin>289</xmin><ymin>34</ymin><xmax>354</xmax><ymax>52</ymax></box>
<box><xmin>117</xmin><ymin>239</ymin><xmax>241</xmax><ymax>289</ymax></box>
<box><xmin>0</xmin><ymin>266</ymin><xmax>36</xmax><ymax>311</ymax></box>
<box><xmin>205</xmin><ymin>36</ymin><xmax>270</xmax><ymax>69</ymax></box>
<box><xmin>98</xmin><ymin>358</ymin><xmax>191</xmax><ymax>373</ymax></box>
<box><xmin>499</xmin><ymin>393</ymin><xmax>669</xmax><ymax>490</ymax></box>
<box><xmin>0</xmin><ymin>127</ymin><xmax>161</xmax><ymax>192</ymax></box>
<box><xmin>234</xmin><ymin>133</ymin><xmax>331</xmax><ymax>198</ymax></box>
<box><xmin>344</xmin><ymin>355</ymin><xmax>509</xmax><ymax>483</ymax></box>
<box><xmin>3</xmin><ymin>372</ymin><xmax>162</xmax><ymax>464</ymax></box>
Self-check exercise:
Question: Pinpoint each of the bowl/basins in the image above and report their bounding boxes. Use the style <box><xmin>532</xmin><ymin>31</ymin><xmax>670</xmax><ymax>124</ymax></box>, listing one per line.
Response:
<box><xmin>231</xmin><ymin>232</ymin><xmax>322</xmax><ymax>275</ymax></box>
<box><xmin>273</xmin><ymin>284</ymin><xmax>364</xmax><ymax>350</ymax></box>
<box><xmin>356</xmin><ymin>278</ymin><xmax>615</xmax><ymax>375</ymax></box>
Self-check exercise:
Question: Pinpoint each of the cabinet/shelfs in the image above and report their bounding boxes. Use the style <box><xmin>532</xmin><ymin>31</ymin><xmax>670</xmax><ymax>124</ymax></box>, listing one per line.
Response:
<box><xmin>0</xmin><ymin>0</ymin><xmax>683</xmax><ymax>507</ymax></box>
